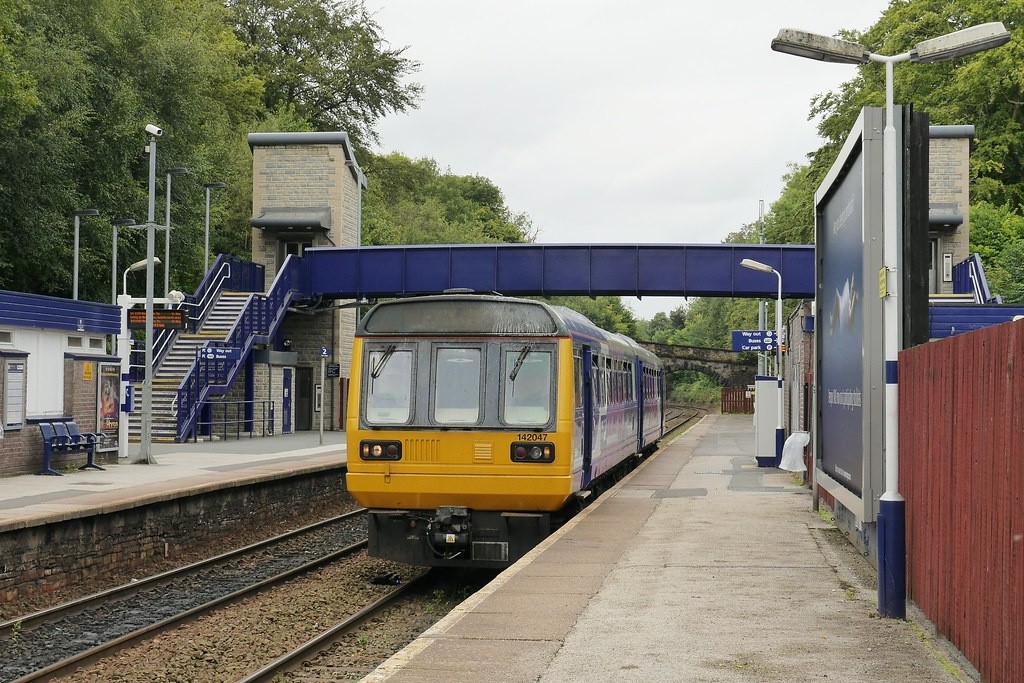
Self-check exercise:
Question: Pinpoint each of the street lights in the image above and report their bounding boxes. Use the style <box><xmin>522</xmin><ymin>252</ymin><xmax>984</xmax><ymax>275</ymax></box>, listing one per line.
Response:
<box><xmin>203</xmin><ymin>182</ymin><xmax>227</xmax><ymax>278</ymax></box>
<box><xmin>164</xmin><ymin>168</ymin><xmax>189</xmax><ymax>296</ymax></box>
<box><xmin>770</xmin><ymin>19</ymin><xmax>1011</xmax><ymax>618</ymax></box>
<box><xmin>119</xmin><ymin>255</ymin><xmax>162</xmax><ymax>459</ymax></box>
<box><xmin>740</xmin><ymin>258</ymin><xmax>783</xmax><ymax>469</ymax></box>
<box><xmin>71</xmin><ymin>208</ymin><xmax>99</xmax><ymax>300</ymax></box>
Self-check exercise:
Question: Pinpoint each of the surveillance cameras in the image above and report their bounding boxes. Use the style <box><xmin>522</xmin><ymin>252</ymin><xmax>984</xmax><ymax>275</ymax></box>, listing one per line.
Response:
<box><xmin>145</xmin><ymin>124</ymin><xmax>163</xmax><ymax>136</ymax></box>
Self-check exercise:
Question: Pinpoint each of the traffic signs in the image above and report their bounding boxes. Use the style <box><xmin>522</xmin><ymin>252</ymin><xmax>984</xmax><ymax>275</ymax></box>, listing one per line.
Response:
<box><xmin>732</xmin><ymin>330</ymin><xmax>777</xmax><ymax>350</ymax></box>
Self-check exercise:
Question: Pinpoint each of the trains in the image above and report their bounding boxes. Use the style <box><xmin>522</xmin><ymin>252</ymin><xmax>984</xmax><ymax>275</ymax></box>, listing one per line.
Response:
<box><xmin>346</xmin><ymin>286</ymin><xmax>665</xmax><ymax>567</ymax></box>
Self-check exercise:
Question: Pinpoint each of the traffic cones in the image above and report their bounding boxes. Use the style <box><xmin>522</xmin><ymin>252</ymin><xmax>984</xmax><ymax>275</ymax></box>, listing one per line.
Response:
<box><xmin>111</xmin><ymin>219</ymin><xmax>136</xmax><ymax>306</ymax></box>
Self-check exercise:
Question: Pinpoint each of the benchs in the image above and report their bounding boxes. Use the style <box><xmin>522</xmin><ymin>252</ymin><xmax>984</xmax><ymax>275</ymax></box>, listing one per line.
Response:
<box><xmin>33</xmin><ymin>422</ymin><xmax>108</xmax><ymax>476</ymax></box>
